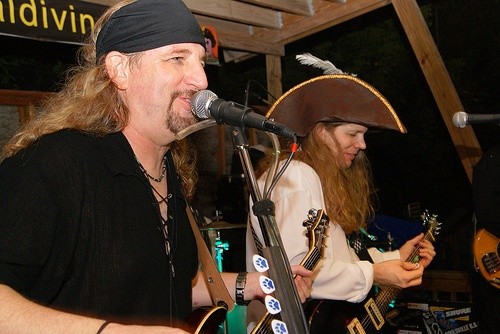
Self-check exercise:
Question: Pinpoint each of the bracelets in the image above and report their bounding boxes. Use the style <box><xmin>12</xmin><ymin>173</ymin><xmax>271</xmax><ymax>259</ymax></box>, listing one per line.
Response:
<box><xmin>235</xmin><ymin>271</ymin><xmax>248</xmax><ymax>305</ymax></box>
<box><xmin>97</xmin><ymin>320</ymin><xmax>112</xmax><ymax>334</ymax></box>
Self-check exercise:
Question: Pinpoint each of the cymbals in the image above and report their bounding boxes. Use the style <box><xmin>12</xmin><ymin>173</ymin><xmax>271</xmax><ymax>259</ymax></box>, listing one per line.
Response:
<box><xmin>199</xmin><ymin>221</ymin><xmax>246</xmax><ymax>229</ymax></box>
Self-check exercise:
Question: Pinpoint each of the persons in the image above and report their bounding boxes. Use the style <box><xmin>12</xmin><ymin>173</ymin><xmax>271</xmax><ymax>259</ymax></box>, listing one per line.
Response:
<box><xmin>245</xmin><ymin>120</ymin><xmax>436</xmax><ymax>334</ymax></box>
<box><xmin>471</xmin><ymin>146</ymin><xmax>500</xmax><ymax>334</ymax></box>
<box><xmin>0</xmin><ymin>0</ymin><xmax>315</xmax><ymax>334</ymax></box>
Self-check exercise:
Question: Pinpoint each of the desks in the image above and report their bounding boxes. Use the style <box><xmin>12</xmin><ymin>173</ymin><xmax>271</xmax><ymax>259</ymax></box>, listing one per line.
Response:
<box><xmin>423</xmin><ymin>270</ymin><xmax>476</xmax><ymax>301</ymax></box>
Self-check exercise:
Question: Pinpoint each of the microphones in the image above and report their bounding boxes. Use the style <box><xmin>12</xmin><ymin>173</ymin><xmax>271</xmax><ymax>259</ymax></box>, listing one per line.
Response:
<box><xmin>190</xmin><ymin>90</ymin><xmax>296</xmax><ymax>141</ymax></box>
<box><xmin>452</xmin><ymin>111</ymin><xmax>500</xmax><ymax>127</ymax></box>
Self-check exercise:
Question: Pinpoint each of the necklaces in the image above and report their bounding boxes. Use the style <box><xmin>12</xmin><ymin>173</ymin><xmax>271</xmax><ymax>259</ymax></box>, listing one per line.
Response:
<box><xmin>138</xmin><ymin>161</ymin><xmax>166</xmax><ymax>182</ymax></box>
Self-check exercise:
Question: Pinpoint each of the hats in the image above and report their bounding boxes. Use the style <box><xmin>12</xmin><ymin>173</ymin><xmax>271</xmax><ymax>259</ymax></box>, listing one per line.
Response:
<box><xmin>264</xmin><ymin>53</ymin><xmax>407</xmax><ymax>150</ymax></box>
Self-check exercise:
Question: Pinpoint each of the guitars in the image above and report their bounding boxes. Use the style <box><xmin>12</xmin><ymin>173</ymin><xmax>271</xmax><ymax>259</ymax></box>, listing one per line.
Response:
<box><xmin>174</xmin><ymin>207</ymin><xmax>331</xmax><ymax>334</ymax></box>
<box><xmin>305</xmin><ymin>208</ymin><xmax>443</xmax><ymax>334</ymax></box>
<box><xmin>473</xmin><ymin>227</ymin><xmax>500</xmax><ymax>288</ymax></box>
